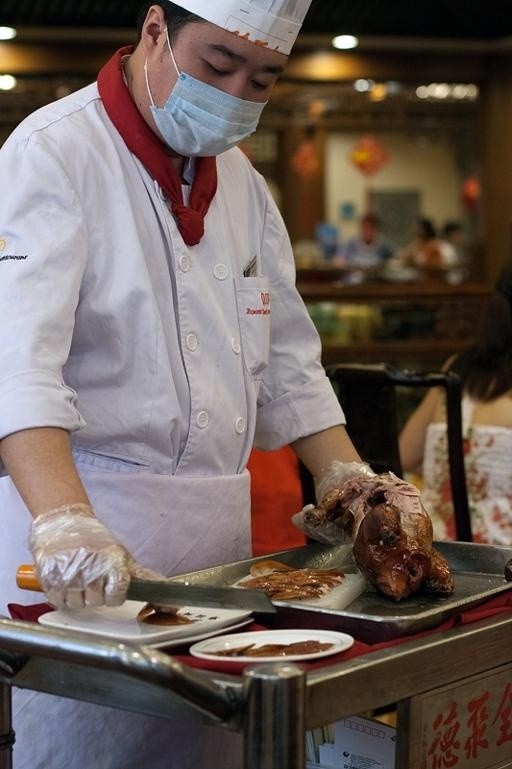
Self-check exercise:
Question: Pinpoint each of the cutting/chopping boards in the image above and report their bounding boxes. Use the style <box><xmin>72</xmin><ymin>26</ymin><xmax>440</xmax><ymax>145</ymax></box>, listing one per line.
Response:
<box><xmin>233</xmin><ymin>561</ymin><xmax>369</xmax><ymax>608</ymax></box>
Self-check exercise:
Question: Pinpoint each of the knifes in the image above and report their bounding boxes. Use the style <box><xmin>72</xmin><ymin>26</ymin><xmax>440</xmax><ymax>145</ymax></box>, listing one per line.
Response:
<box><xmin>14</xmin><ymin>560</ymin><xmax>276</xmax><ymax>618</ymax></box>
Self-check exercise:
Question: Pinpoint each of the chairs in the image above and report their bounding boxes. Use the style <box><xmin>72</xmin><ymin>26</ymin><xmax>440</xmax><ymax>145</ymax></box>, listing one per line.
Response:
<box><xmin>297</xmin><ymin>363</ymin><xmax>473</xmax><ymax>545</ymax></box>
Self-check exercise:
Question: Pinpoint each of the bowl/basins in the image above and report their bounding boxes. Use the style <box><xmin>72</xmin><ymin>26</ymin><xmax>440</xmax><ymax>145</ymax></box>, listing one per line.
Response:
<box><xmin>303</xmin><ymin>306</ymin><xmax>372</xmax><ymax>361</ymax></box>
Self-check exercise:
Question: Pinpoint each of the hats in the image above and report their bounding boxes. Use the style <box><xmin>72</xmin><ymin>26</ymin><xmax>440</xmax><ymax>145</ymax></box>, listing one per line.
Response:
<box><xmin>170</xmin><ymin>0</ymin><xmax>312</xmax><ymax>59</ymax></box>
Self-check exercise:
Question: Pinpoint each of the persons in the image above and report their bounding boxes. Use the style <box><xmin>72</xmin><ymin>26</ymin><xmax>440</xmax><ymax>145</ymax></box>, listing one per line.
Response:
<box><xmin>0</xmin><ymin>0</ymin><xmax>380</xmax><ymax>766</ymax></box>
<box><xmin>439</xmin><ymin>220</ymin><xmax>466</xmax><ymax>263</ymax></box>
<box><xmin>341</xmin><ymin>212</ymin><xmax>396</xmax><ymax>275</ymax></box>
<box><xmin>395</xmin><ymin>252</ymin><xmax>510</xmax><ymax>553</ymax></box>
<box><xmin>385</xmin><ymin>216</ymin><xmax>459</xmax><ymax>277</ymax></box>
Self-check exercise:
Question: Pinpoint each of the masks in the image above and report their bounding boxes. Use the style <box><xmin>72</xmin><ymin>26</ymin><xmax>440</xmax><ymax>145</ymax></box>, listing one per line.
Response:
<box><xmin>143</xmin><ymin>28</ymin><xmax>269</xmax><ymax>159</ymax></box>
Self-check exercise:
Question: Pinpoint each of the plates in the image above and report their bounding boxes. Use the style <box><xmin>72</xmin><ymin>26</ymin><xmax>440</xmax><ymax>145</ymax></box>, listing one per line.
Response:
<box><xmin>189</xmin><ymin>628</ymin><xmax>354</xmax><ymax>665</ymax></box>
<box><xmin>37</xmin><ymin>607</ymin><xmax>257</xmax><ymax>648</ymax></box>
